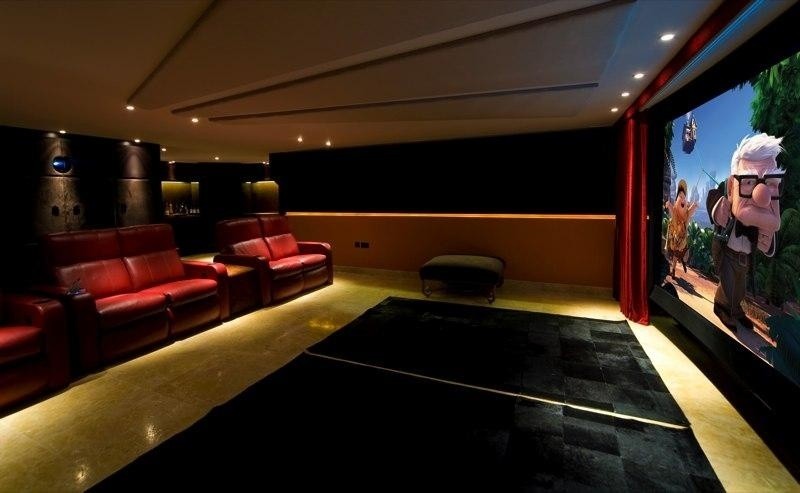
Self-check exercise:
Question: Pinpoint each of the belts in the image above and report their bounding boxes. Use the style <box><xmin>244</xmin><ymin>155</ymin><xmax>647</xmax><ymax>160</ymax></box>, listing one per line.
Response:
<box><xmin>724</xmin><ymin>248</ymin><xmax>753</xmax><ymax>267</ymax></box>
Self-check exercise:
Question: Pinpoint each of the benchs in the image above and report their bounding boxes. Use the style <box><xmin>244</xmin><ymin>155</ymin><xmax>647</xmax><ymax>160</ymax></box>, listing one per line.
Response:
<box><xmin>418</xmin><ymin>252</ymin><xmax>508</xmax><ymax>305</ymax></box>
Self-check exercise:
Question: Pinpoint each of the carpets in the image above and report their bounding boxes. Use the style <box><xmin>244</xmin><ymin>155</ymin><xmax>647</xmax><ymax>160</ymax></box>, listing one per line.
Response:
<box><xmin>306</xmin><ymin>292</ymin><xmax>697</xmax><ymax>426</ymax></box>
<box><xmin>83</xmin><ymin>349</ymin><xmax>728</xmax><ymax>491</ymax></box>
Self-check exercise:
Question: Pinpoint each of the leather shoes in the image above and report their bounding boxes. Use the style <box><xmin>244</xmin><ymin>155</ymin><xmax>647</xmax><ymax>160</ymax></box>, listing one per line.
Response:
<box><xmin>737</xmin><ymin>303</ymin><xmax>755</xmax><ymax>331</ymax></box>
<box><xmin>713</xmin><ymin>302</ymin><xmax>737</xmax><ymax>332</ymax></box>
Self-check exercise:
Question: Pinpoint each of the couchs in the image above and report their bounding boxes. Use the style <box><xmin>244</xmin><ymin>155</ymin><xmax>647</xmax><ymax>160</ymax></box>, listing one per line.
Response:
<box><xmin>213</xmin><ymin>213</ymin><xmax>336</xmax><ymax>310</ymax></box>
<box><xmin>0</xmin><ymin>295</ymin><xmax>74</xmax><ymax>412</ymax></box>
<box><xmin>45</xmin><ymin>222</ymin><xmax>230</xmax><ymax>379</ymax></box>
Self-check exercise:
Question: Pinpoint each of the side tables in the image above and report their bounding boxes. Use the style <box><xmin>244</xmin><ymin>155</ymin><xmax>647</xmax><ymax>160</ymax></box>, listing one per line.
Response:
<box><xmin>220</xmin><ymin>262</ymin><xmax>261</xmax><ymax>316</ymax></box>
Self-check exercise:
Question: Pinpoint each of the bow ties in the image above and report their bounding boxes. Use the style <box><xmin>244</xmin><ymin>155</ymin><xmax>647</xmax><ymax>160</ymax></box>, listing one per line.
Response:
<box><xmin>735</xmin><ymin>219</ymin><xmax>759</xmax><ymax>243</ymax></box>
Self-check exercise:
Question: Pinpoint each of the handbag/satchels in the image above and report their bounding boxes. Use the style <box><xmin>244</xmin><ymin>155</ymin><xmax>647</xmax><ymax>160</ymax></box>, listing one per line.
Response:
<box><xmin>674</xmin><ymin>222</ymin><xmax>686</xmax><ymax>247</ymax></box>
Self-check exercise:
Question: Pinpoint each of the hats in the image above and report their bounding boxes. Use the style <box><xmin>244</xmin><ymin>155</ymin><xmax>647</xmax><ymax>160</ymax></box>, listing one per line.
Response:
<box><xmin>678</xmin><ymin>180</ymin><xmax>687</xmax><ymax>196</ymax></box>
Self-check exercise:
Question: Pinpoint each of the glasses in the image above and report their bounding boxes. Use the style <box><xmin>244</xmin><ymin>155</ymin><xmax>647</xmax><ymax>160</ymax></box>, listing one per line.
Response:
<box><xmin>734</xmin><ymin>174</ymin><xmax>785</xmax><ymax>200</ymax></box>
